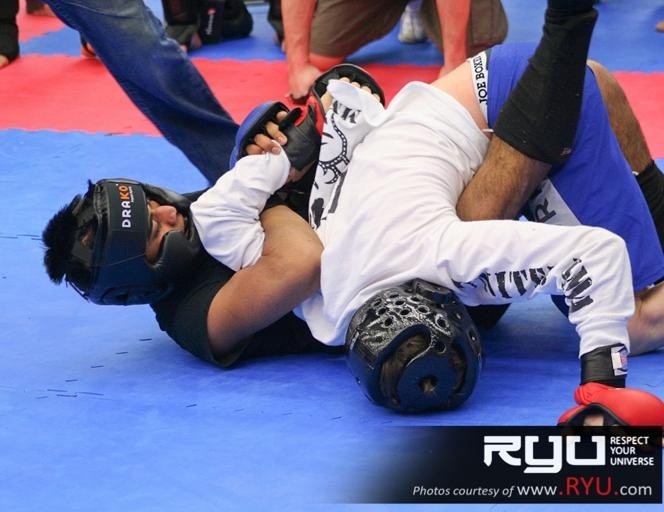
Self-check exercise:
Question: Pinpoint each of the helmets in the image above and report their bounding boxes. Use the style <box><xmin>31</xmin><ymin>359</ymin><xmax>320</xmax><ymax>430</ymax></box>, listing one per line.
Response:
<box><xmin>346</xmin><ymin>280</ymin><xmax>482</xmax><ymax>413</ymax></box>
<box><xmin>66</xmin><ymin>177</ymin><xmax>205</xmax><ymax>306</ymax></box>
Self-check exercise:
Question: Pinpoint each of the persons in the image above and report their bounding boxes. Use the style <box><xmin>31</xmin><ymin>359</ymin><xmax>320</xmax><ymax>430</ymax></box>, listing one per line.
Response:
<box><xmin>43</xmin><ymin>0</ymin><xmax>240</xmax><ymax>185</ymax></box>
<box><xmin>0</xmin><ymin>0</ymin><xmax>287</xmax><ymax>69</ymax></box>
<box><xmin>279</xmin><ymin>0</ymin><xmax>509</xmax><ymax>105</ymax></box>
<box><xmin>43</xmin><ymin>0</ymin><xmax>664</xmax><ymax>367</ymax></box>
<box><xmin>188</xmin><ymin>41</ymin><xmax>664</xmax><ymax>427</ymax></box>
